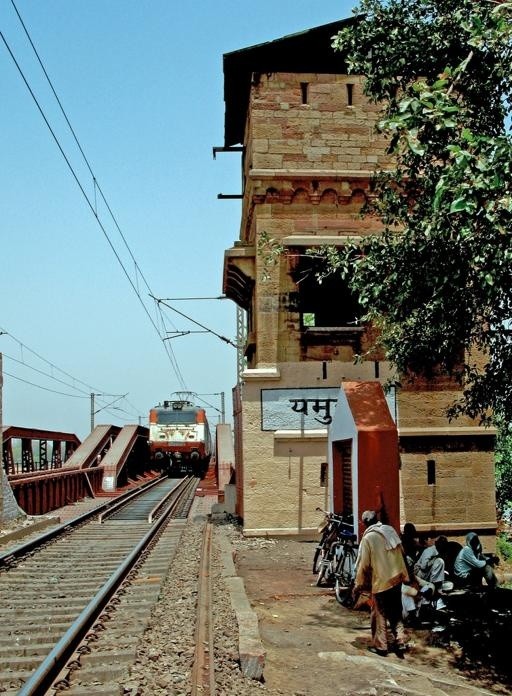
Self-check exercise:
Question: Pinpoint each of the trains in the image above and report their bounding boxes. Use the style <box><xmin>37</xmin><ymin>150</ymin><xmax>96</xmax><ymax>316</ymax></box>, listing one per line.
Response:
<box><xmin>148</xmin><ymin>400</ymin><xmax>213</xmax><ymax>478</ymax></box>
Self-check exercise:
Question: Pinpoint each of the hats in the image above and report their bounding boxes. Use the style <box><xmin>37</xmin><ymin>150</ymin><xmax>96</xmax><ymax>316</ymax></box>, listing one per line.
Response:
<box><xmin>317</xmin><ymin>521</ymin><xmax>328</xmax><ymax>534</ymax></box>
<box><xmin>362</xmin><ymin>511</ymin><xmax>376</xmax><ymax>524</ymax></box>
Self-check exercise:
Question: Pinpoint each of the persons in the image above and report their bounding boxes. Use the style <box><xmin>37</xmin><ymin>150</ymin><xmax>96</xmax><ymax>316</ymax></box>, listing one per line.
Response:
<box><xmin>401</xmin><ymin>572</ymin><xmax>435</xmax><ymax>626</ymax></box>
<box><xmin>415</xmin><ymin>536</ymin><xmax>448</xmax><ymax>595</ymax></box>
<box><xmin>453</xmin><ymin>533</ymin><xmax>490</xmax><ymax>584</ymax></box>
<box><xmin>352</xmin><ymin>510</ymin><xmax>409</xmax><ymax>657</ymax></box>
<box><xmin>401</xmin><ymin>523</ymin><xmax>422</xmax><ymax>560</ymax></box>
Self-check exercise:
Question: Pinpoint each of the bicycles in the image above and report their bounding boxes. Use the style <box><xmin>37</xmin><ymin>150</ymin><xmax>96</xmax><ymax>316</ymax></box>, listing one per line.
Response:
<box><xmin>315</xmin><ymin>518</ymin><xmax>359</xmax><ymax>606</ymax></box>
<box><xmin>312</xmin><ymin>506</ymin><xmax>358</xmax><ymax>595</ymax></box>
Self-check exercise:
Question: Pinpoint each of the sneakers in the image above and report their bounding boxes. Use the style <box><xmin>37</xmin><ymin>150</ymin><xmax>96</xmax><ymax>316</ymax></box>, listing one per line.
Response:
<box><xmin>368</xmin><ymin>646</ymin><xmax>387</xmax><ymax>655</ymax></box>
<box><xmin>391</xmin><ymin>644</ymin><xmax>407</xmax><ymax>651</ymax></box>
